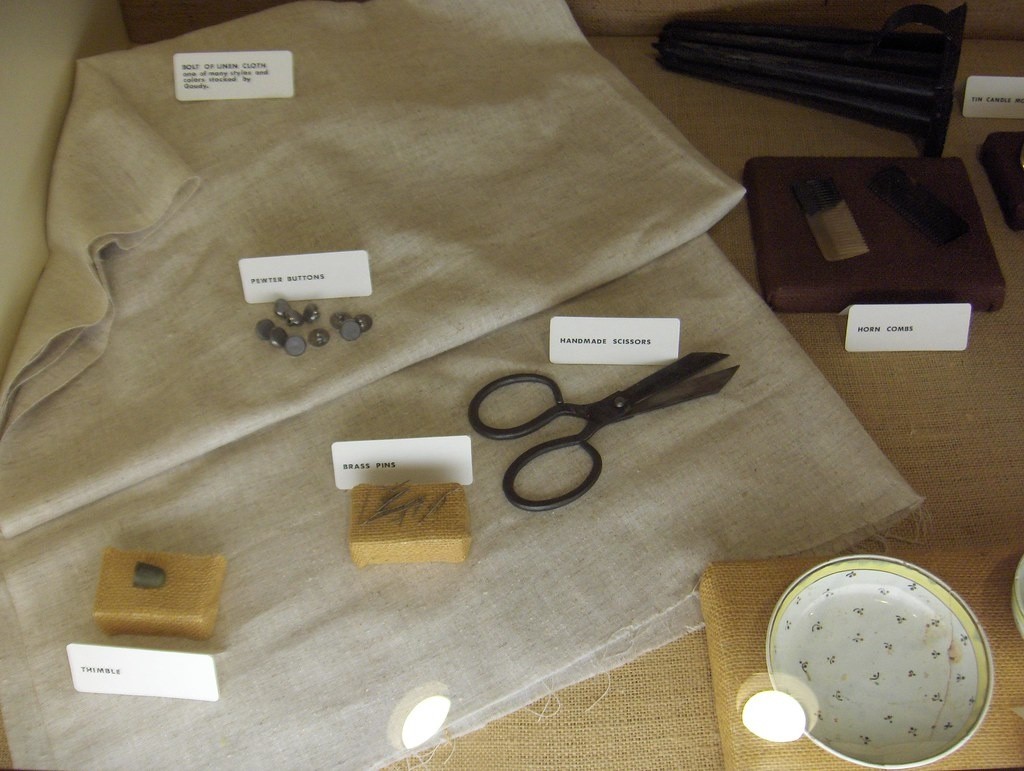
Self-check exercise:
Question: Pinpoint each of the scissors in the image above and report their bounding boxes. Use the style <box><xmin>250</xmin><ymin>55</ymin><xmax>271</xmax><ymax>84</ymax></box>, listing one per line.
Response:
<box><xmin>466</xmin><ymin>346</ymin><xmax>741</xmax><ymax>511</ymax></box>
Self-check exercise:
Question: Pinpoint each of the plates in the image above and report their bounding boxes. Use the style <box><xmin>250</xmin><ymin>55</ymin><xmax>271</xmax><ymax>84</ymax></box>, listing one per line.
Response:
<box><xmin>765</xmin><ymin>554</ymin><xmax>994</xmax><ymax>770</ymax></box>
